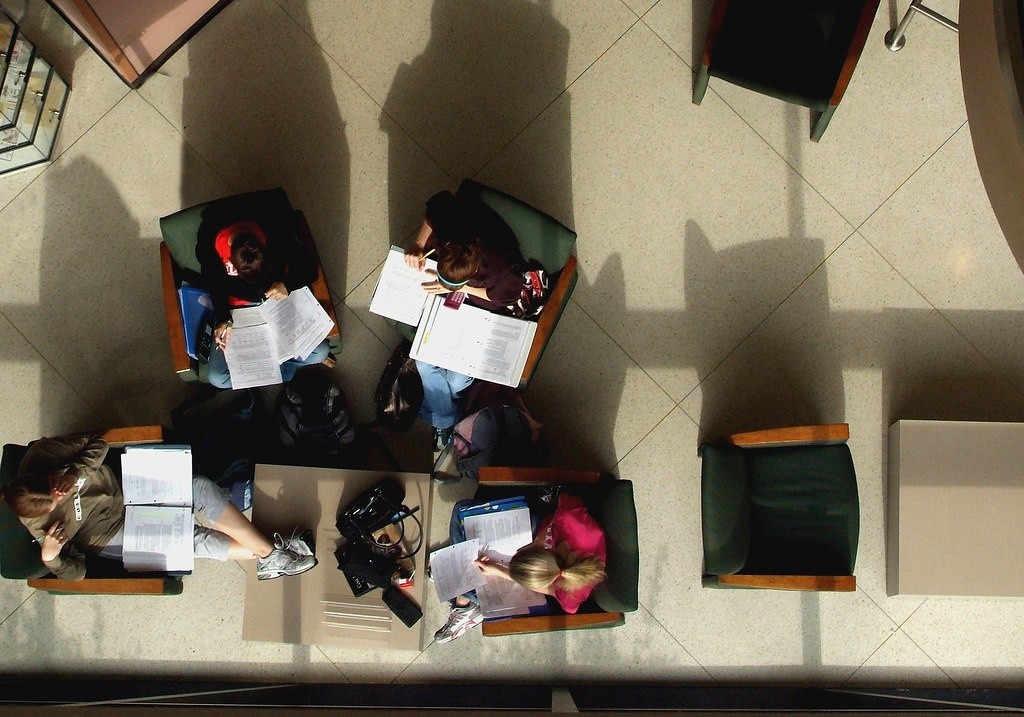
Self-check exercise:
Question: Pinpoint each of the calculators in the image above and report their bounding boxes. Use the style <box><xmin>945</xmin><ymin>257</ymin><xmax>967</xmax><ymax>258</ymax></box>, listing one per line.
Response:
<box><xmin>443</xmin><ymin>291</ymin><xmax>464</xmax><ymax>310</ymax></box>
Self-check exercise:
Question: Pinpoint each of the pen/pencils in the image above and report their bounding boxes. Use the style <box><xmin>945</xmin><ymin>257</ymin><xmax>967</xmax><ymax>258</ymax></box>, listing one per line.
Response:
<box><xmin>393</xmin><ymin>507</ymin><xmax>420</xmax><ymax>524</ymax></box>
<box><xmin>419</xmin><ymin>248</ymin><xmax>435</xmax><ymax>261</ymax></box>
<box><xmin>32</xmin><ymin>527</ymin><xmax>65</xmax><ymax>542</ymax></box>
<box><xmin>216</xmin><ymin>318</ymin><xmax>232</xmax><ymax>350</ymax></box>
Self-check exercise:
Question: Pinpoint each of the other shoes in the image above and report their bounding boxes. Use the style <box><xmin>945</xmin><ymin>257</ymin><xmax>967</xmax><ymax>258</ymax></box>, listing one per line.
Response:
<box><xmin>321</xmin><ymin>351</ymin><xmax>337</xmax><ymax>368</ymax></box>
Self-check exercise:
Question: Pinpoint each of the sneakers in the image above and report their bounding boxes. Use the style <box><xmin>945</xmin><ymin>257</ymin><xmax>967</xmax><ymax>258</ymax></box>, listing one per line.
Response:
<box><xmin>255</xmin><ymin>525</ymin><xmax>318</xmax><ymax>581</ymax></box>
<box><xmin>434</xmin><ymin>602</ymin><xmax>485</xmax><ymax>645</ymax></box>
<box><xmin>432</xmin><ymin>425</ymin><xmax>453</xmax><ymax>452</ymax></box>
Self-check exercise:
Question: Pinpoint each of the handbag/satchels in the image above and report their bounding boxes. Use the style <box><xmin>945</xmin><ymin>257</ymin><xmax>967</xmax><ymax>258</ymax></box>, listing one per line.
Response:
<box><xmin>168</xmin><ymin>383</ymin><xmax>264</xmax><ymax>446</ymax></box>
<box><xmin>335</xmin><ymin>476</ymin><xmax>425</xmax><ymax>560</ymax></box>
<box><xmin>374</xmin><ymin>344</ymin><xmax>424</xmax><ymax>433</ymax></box>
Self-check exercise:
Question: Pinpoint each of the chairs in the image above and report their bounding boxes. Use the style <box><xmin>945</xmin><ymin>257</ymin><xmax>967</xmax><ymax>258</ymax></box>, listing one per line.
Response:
<box><xmin>160</xmin><ymin>188</ymin><xmax>344</xmax><ymax>385</ymax></box>
<box><xmin>394</xmin><ymin>181</ymin><xmax>581</xmax><ymax>390</ymax></box>
<box><xmin>694</xmin><ymin>424</ymin><xmax>860</xmax><ymax>591</ymax></box>
<box><xmin>0</xmin><ymin>426</ymin><xmax>187</xmax><ymax>595</ymax></box>
<box><xmin>473</xmin><ymin>467</ymin><xmax>641</xmax><ymax>637</ymax></box>
<box><xmin>45</xmin><ymin>0</ymin><xmax>232</xmax><ymax>89</ymax></box>
<box><xmin>693</xmin><ymin>0</ymin><xmax>883</xmax><ymax>142</ymax></box>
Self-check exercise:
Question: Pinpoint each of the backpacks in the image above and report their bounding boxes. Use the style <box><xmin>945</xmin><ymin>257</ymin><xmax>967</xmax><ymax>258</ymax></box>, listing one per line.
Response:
<box><xmin>431</xmin><ymin>381</ymin><xmax>542</xmax><ymax>483</ymax></box>
<box><xmin>274</xmin><ymin>380</ymin><xmax>357</xmax><ymax>449</ymax></box>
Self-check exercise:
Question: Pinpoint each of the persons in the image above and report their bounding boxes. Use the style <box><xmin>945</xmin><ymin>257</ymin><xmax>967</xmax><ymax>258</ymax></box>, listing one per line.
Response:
<box><xmin>427</xmin><ymin>493</ymin><xmax>606</xmax><ymax>644</ymax></box>
<box><xmin>404</xmin><ymin>189</ymin><xmax>523</xmax><ymax>453</ymax></box>
<box><xmin>195</xmin><ymin>207</ymin><xmax>336</xmax><ymax>389</ymax></box>
<box><xmin>0</xmin><ymin>433</ymin><xmax>318</xmax><ymax>583</ymax></box>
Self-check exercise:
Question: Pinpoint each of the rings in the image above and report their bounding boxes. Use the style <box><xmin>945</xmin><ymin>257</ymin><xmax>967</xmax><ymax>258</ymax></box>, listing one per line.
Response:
<box><xmin>59</xmin><ymin>535</ymin><xmax>63</xmax><ymax>539</ymax></box>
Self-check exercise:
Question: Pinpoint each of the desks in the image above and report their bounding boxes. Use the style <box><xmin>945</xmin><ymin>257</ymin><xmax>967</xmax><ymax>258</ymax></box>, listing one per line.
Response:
<box><xmin>244</xmin><ymin>465</ymin><xmax>433</xmax><ymax>650</ymax></box>
<box><xmin>886</xmin><ymin>420</ymin><xmax>1024</xmax><ymax>597</ymax></box>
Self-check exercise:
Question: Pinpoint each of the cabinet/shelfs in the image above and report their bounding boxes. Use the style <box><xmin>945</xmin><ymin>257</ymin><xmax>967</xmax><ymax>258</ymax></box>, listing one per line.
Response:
<box><xmin>0</xmin><ymin>11</ymin><xmax>72</xmax><ymax>173</ymax></box>
